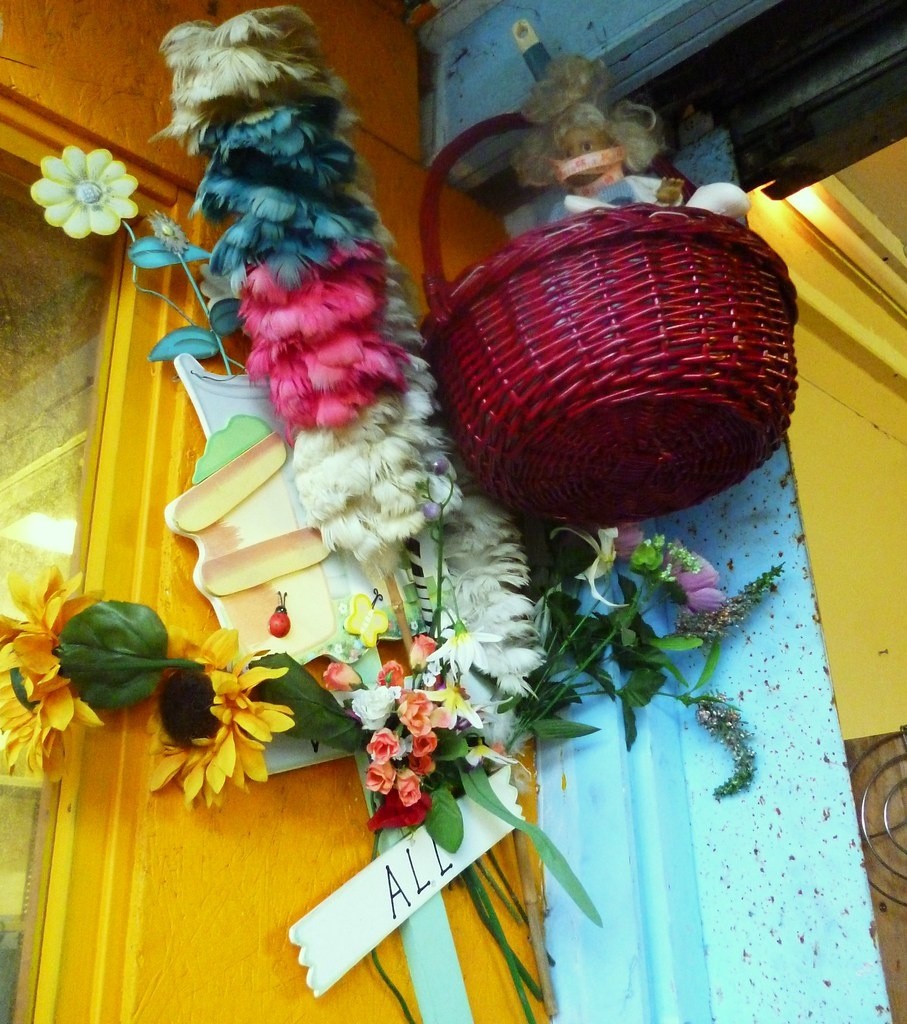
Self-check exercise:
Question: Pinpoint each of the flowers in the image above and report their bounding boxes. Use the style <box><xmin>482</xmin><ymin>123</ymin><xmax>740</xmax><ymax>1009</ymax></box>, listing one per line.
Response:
<box><xmin>0</xmin><ymin>143</ymin><xmax>783</xmax><ymax>1023</ymax></box>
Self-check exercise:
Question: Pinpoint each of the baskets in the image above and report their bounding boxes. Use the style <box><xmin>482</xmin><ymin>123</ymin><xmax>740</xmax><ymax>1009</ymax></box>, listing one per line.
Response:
<box><xmin>421</xmin><ymin>114</ymin><xmax>799</xmax><ymax>532</ymax></box>
